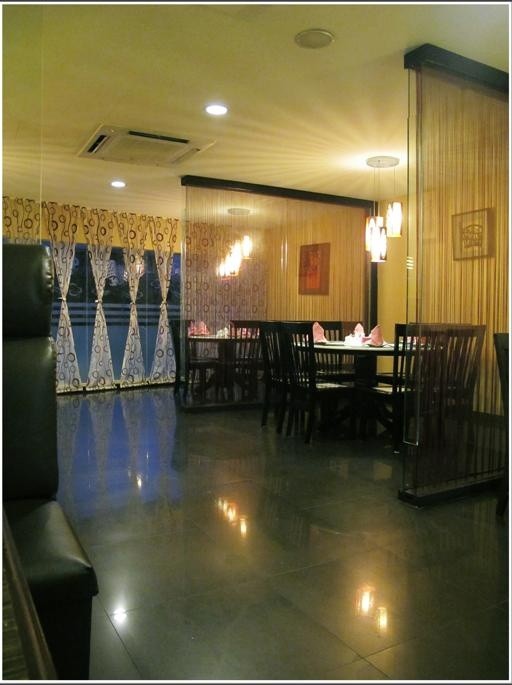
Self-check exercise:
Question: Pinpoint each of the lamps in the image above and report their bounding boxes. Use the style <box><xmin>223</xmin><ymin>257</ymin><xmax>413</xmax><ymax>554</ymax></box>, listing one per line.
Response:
<box><xmin>221</xmin><ymin>208</ymin><xmax>251</xmax><ymax>280</ymax></box>
<box><xmin>364</xmin><ymin>155</ymin><xmax>402</xmax><ymax>262</ymax></box>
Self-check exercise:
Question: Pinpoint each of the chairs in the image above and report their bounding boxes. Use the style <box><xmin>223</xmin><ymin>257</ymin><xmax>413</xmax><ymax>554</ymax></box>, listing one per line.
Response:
<box><xmin>294</xmin><ymin>322</ymin><xmax>355</xmax><ymax>386</ymax></box>
<box><xmin>493</xmin><ymin>333</ymin><xmax>508</xmax><ymax>516</ymax></box>
<box><xmin>256</xmin><ymin>323</ymin><xmax>326</xmax><ymax>435</ymax></box>
<box><xmin>3</xmin><ymin>244</ymin><xmax>99</xmax><ymax>680</ymax></box>
<box><xmin>284</xmin><ymin>321</ymin><xmax>358</xmax><ymax>443</ymax></box>
<box><xmin>365</xmin><ymin>323</ymin><xmax>443</xmax><ymax>455</ymax></box>
<box><xmin>170</xmin><ymin>319</ymin><xmax>283</xmax><ymax>413</ymax></box>
<box><xmin>373</xmin><ymin>322</ymin><xmax>428</xmax><ymax>387</ymax></box>
<box><xmin>411</xmin><ymin>324</ymin><xmax>486</xmax><ymax>444</ymax></box>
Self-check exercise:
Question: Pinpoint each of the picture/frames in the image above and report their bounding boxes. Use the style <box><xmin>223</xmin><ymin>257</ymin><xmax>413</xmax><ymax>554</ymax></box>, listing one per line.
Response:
<box><xmin>451</xmin><ymin>208</ymin><xmax>494</xmax><ymax>260</ymax></box>
<box><xmin>298</xmin><ymin>242</ymin><xmax>330</xmax><ymax>295</ymax></box>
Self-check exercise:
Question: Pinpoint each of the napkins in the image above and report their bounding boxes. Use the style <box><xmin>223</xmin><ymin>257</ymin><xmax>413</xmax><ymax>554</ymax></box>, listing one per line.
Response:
<box><xmin>350</xmin><ymin>323</ymin><xmax>365</xmax><ymax>340</ymax></box>
<box><xmin>401</xmin><ymin>334</ymin><xmax>425</xmax><ymax>345</ymax></box>
<box><xmin>364</xmin><ymin>325</ymin><xmax>383</xmax><ymax>345</ymax></box>
<box><xmin>302</xmin><ymin>322</ymin><xmax>327</xmax><ymax>343</ymax></box>
